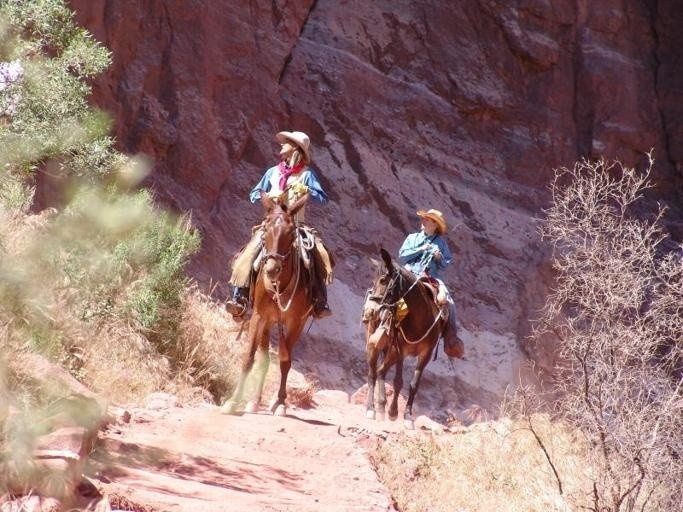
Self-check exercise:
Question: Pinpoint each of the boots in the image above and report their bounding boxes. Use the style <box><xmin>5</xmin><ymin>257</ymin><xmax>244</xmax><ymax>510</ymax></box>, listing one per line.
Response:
<box><xmin>310</xmin><ymin>266</ymin><xmax>333</xmax><ymax>321</ymax></box>
<box><xmin>224</xmin><ymin>283</ymin><xmax>248</xmax><ymax>324</ymax></box>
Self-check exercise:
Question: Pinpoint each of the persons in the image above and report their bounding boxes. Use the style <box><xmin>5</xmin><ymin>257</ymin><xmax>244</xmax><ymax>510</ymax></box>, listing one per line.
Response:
<box><xmin>223</xmin><ymin>130</ymin><xmax>332</xmax><ymax>325</ymax></box>
<box><xmin>396</xmin><ymin>208</ymin><xmax>464</xmax><ymax>359</ymax></box>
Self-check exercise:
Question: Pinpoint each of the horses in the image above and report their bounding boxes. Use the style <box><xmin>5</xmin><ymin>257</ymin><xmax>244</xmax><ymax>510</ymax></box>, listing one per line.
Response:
<box><xmin>363</xmin><ymin>248</ymin><xmax>445</xmax><ymax>430</ymax></box>
<box><xmin>220</xmin><ymin>188</ymin><xmax>319</xmax><ymax>415</ymax></box>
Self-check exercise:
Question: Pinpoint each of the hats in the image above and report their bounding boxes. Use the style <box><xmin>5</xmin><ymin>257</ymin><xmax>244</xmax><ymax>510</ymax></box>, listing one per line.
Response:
<box><xmin>272</xmin><ymin>129</ymin><xmax>311</xmax><ymax>164</ymax></box>
<box><xmin>416</xmin><ymin>207</ymin><xmax>446</xmax><ymax>233</ymax></box>
